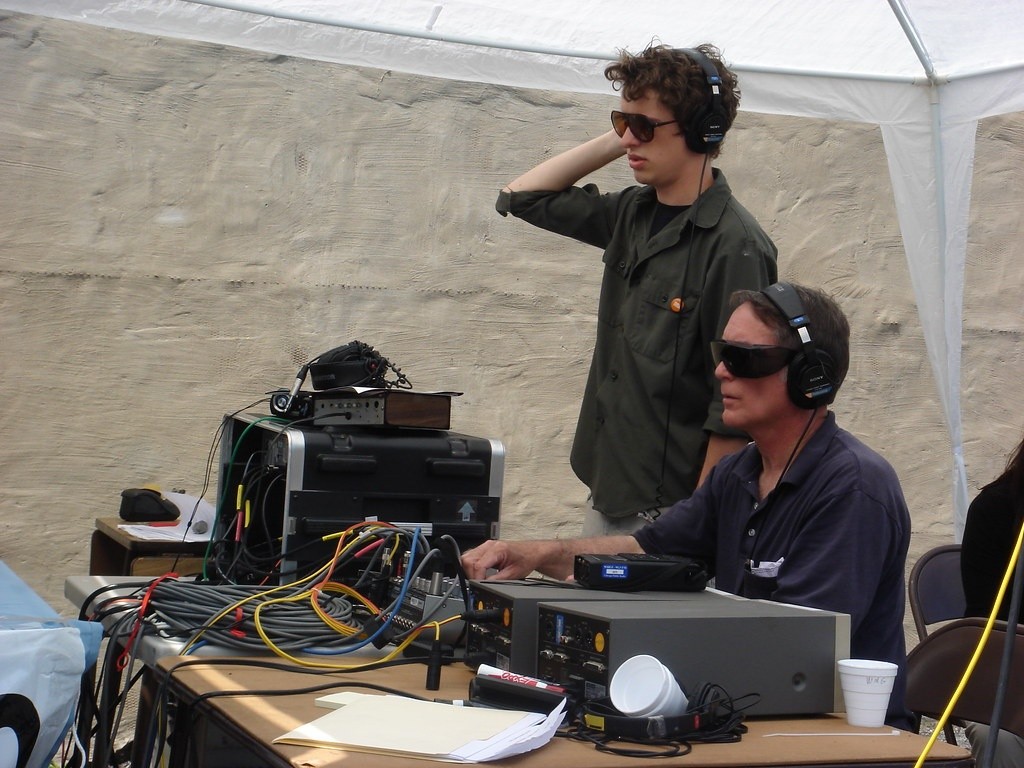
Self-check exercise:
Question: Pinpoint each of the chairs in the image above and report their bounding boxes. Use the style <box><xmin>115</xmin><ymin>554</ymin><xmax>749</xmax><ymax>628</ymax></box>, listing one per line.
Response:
<box><xmin>907</xmin><ymin>616</ymin><xmax>1024</xmax><ymax>736</ymax></box>
<box><xmin>908</xmin><ymin>544</ymin><xmax>967</xmax><ymax>745</ymax></box>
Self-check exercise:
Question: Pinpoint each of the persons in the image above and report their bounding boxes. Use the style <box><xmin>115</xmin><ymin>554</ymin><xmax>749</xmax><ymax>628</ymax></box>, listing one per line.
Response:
<box><xmin>960</xmin><ymin>437</ymin><xmax>1024</xmax><ymax>768</ymax></box>
<box><xmin>495</xmin><ymin>34</ymin><xmax>777</xmax><ymax>539</ymax></box>
<box><xmin>460</xmin><ymin>282</ymin><xmax>914</xmax><ymax>732</ymax></box>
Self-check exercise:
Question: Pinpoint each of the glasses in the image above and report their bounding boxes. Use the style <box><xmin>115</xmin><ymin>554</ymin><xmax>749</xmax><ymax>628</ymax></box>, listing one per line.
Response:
<box><xmin>710</xmin><ymin>341</ymin><xmax>800</xmax><ymax>378</ymax></box>
<box><xmin>611</xmin><ymin>111</ymin><xmax>678</xmax><ymax>142</ymax></box>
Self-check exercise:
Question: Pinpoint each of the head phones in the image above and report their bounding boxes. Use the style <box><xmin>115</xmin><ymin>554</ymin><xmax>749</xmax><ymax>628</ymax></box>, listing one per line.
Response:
<box><xmin>582</xmin><ymin>682</ymin><xmax>744</xmax><ymax>743</ymax></box>
<box><xmin>673</xmin><ymin>48</ymin><xmax>727</xmax><ymax>154</ymax></box>
<box><xmin>310</xmin><ymin>340</ymin><xmax>387</xmax><ymax>390</ymax></box>
<box><xmin>760</xmin><ymin>281</ymin><xmax>840</xmax><ymax>410</ymax></box>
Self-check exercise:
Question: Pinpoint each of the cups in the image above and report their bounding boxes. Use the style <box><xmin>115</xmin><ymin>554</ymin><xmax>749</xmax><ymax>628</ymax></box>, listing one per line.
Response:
<box><xmin>610</xmin><ymin>655</ymin><xmax>689</xmax><ymax>718</ymax></box>
<box><xmin>838</xmin><ymin>659</ymin><xmax>898</xmax><ymax>727</ymax></box>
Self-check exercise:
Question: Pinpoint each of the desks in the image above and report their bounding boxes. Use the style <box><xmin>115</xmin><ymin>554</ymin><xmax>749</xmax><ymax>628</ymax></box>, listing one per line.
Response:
<box><xmin>62</xmin><ymin>575</ymin><xmax>402</xmax><ymax>768</ymax></box>
<box><xmin>89</xmin><ymin>517</ymin><xmax>215</xmax><ymax>577</ymax></box>
<box><xmin>156</xmin><ymin>656</ymin><xmax>971</xmax><ymax>768</ymax></box>
